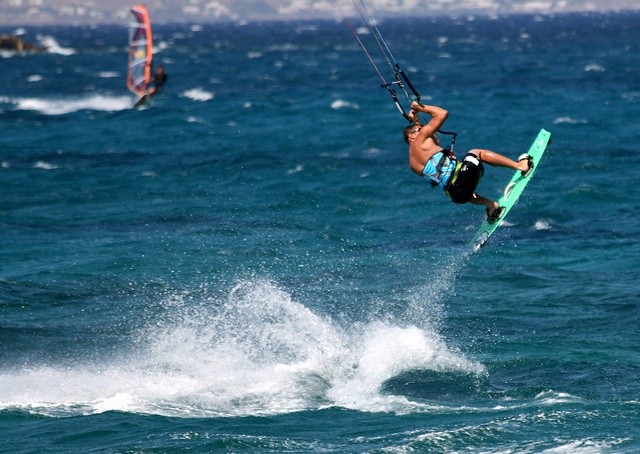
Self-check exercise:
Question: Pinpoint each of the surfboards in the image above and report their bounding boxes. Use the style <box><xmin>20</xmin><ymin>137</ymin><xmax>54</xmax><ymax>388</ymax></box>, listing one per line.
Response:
<box><xmin>467</xmin><ymin>128</ymin><xmax>552</xmax><ymax>254</ymax></box>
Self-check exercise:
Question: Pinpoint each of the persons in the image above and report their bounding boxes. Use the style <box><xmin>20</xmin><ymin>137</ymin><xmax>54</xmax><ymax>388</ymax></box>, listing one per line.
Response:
<box><xmin>148</xmin><ymin>64</ymin><xmax>165</xmax><ymax>98</ymax></box>
<box><xmin>402</xmin><ymin>99</ymin><xmax>534</xmax><ymax>223</ymax></box>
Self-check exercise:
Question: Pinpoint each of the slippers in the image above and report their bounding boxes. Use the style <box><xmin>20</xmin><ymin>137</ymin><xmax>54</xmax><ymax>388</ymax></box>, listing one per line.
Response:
<box><xmin>486</xmin><ymin>205</ymin><xmax>506</xmax><ymax>224</ymax></box>
<box><xmin>518</xmin><ymin>153</ymin><xmax>533</xmax><ymax>178</ymax></box>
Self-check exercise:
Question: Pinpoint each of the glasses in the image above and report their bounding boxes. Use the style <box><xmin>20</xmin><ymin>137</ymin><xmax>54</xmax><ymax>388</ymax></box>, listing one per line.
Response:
<box><xmin>409</xmin><ymin>127</ymin><xmax>421</xmax><ymax>134</ymax></box>
